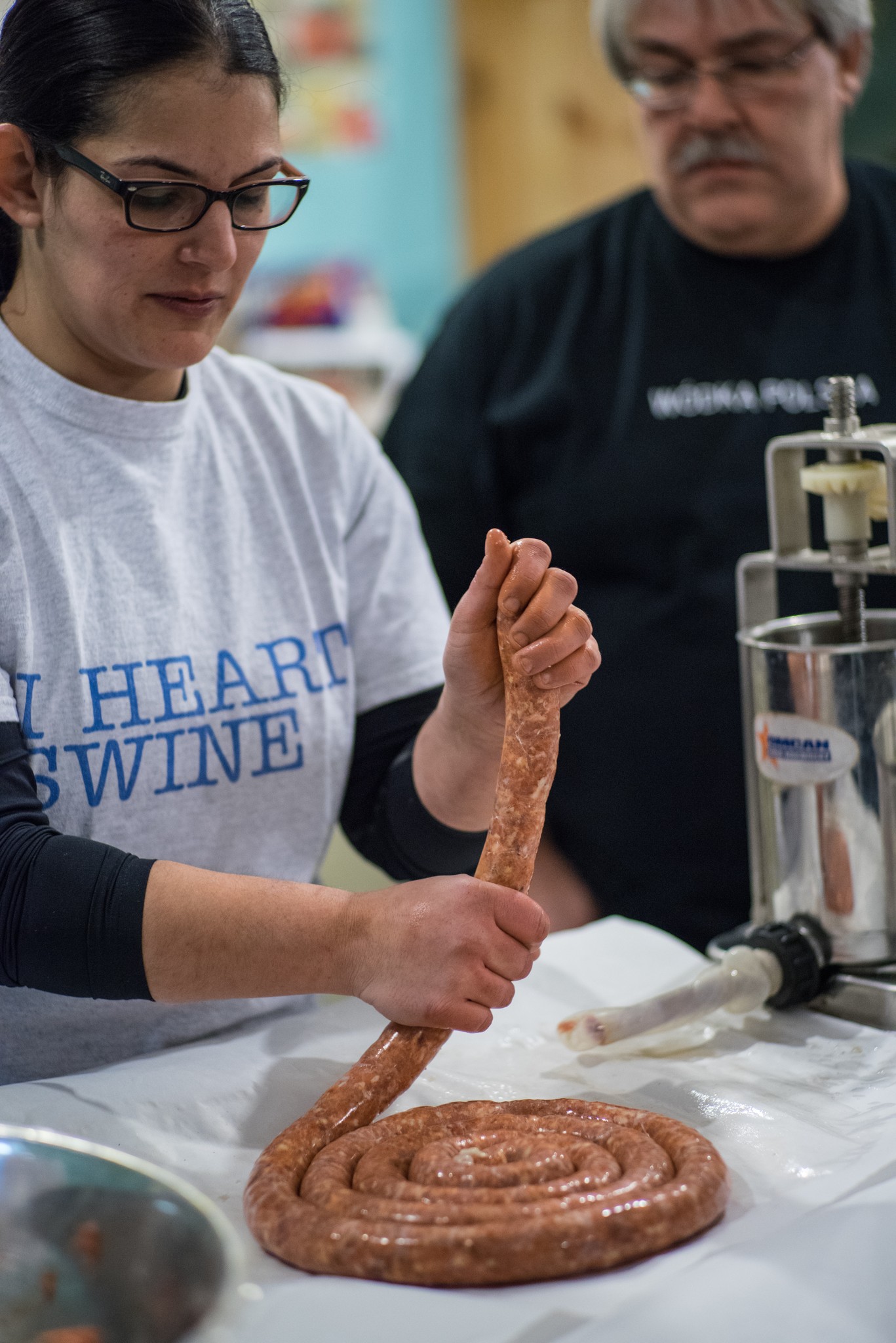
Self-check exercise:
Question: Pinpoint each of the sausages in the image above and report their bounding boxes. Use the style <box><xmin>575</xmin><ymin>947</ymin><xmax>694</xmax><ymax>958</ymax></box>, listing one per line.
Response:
<box><xmin>240</xmin><ymin>609</ymin><xmax>732</xmax><ymax>1285</ymax></box>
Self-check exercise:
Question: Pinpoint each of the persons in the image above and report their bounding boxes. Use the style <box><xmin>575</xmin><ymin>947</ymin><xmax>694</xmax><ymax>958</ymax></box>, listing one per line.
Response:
<box><xmin>376</xmin><ymin>0</ymin><xmax>896</xmax><ymax>956</ymax></box>
<box><xmin>0</xmin><ymin>1</ymin><xmax>601</xmax><ymax>1087</ymax></box>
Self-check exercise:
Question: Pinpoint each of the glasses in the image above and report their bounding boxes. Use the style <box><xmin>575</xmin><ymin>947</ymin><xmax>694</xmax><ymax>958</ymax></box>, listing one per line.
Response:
<box><xmin>20</xmin><ymin>119</ymin><xmax>311</xmax><ymax>230</ymax></box>
<box><xmin>622</xmin><ymin>28</ymin><xmax>824</xmax><ymax>112</ymax></box>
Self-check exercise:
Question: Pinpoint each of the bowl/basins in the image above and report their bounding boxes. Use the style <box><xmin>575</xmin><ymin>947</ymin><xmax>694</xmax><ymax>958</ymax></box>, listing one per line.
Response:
<box><xmin>2</xmin><ymin>1125</ymin><xmax>242</xmax><ymax>1343</ymax></box>
